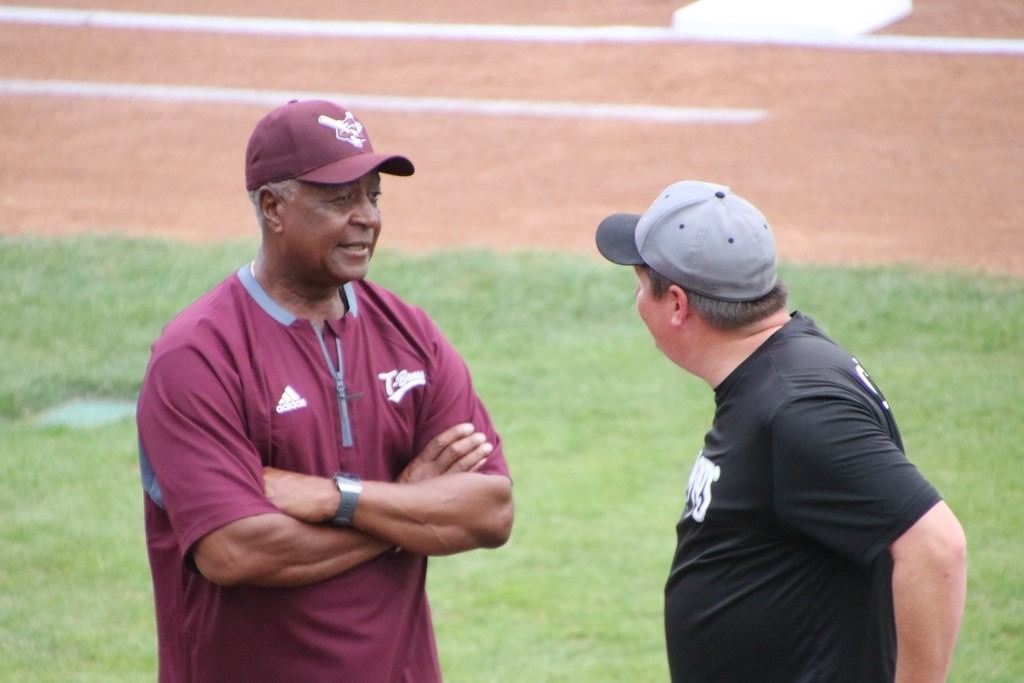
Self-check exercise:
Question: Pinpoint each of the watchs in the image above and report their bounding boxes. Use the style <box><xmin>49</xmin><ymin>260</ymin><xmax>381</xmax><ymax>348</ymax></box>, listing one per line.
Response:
<box><xmin>330</xmin><ymin>472</ymin><xmax>361</xmax><ymax>526</ymax></box>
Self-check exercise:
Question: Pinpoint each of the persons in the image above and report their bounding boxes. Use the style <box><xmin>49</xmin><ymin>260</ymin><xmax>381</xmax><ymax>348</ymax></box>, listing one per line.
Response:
<box><xmin>596</xmin><ymin>179</ymin><xmax>967</xmax><ymax>683</ymax></box>
<box><xmin>137</xmin><ymin>100</ymin><xmax>515</xmax><ymax>683</ymax></box>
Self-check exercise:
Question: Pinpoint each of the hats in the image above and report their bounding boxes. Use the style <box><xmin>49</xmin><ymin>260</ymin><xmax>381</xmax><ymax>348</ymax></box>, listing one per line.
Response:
<box><xmin>595</xmin><ymin>178</ymin><xmax>776</xmax><ymax>302</ymax></box>
<box><xmin>246</xmin><ymin>99</ymin><xmax>413</xmax><ymax>195</ymax></box>
